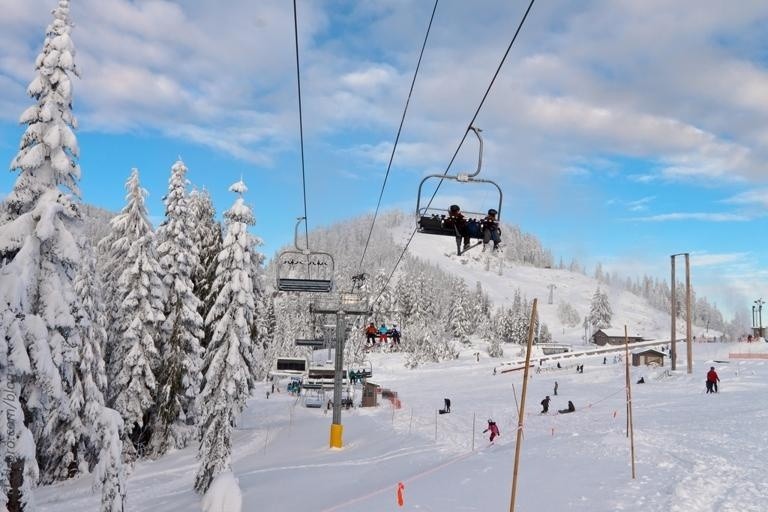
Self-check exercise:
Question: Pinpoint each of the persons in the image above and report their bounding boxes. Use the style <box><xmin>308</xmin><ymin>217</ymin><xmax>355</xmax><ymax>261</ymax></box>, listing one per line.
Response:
<box><xmin>390</xmin><ymin>324</ymin><xmax>401</xmax><ymax>344</ymax></box>
<box><xmin>636</xmin><ymin>376</ymin><xmax>644</xmax><ymax>384</ymax></box>
<box><xmin>264</xmin><ymin>378</ymin><xmax>303</xmax><ymax>399</ymax></box>
<box><xmin>706</xmin><ymin>380</ymin><xmax>714</xmax><ymax>393</ymax></box>
<box><xmin>706</xmin><ymin>366</ymin><xmax>720</xmax><ymax>393</ymax></box>
<box><xmin>443</xmin><ymin>398</ymin><xmax>451</xmax><ymax>412</ymax></box>
<box><xmin>349</xmin><ymin>368</ymin><xmax>367</xmax><ymax>386</ymax></box>
<box><xmin>483</xmin><ymin>419</ymin><xmax>500</xmax><ymax>442</ymax></box>
<box><xmin>540</xmin><ymin>396</ymin><xmax>550</xmax><ymax>413</ymax></box>
<box><xmin>747</xmin><ymin>335</ymin><xmax>751</xmax><ymax>343</ymax></box>
<box><xmin>554</xmin><ymin>381</ymin><xmax>558</xmax><ymax>395</ymax></box>
<box><xmin>448</xmin><ymin>205</ymin><xmax>470</xmax><ymax>254</ymax></box>
<box><xmin>557</xmin><ymin>400</ymin><xmax>575</xmax><ymax>413</ymax></box>
<box><xmin>367</xmin><ymin>322</ymin><xmax>378</xmax><ymax>344</ymax></box>
<box><xmin>480</xmin><ymin>208</ymin><xmax>501</xmax><ymax>252</ymax></box>
<box><xmin>377</xmin><ymin>323</ymin><xmax>389</xmax><ymax>343</ymax></box>
<box><xmin>326</xmin><ymin>396</ymin><xmax>354</xmax><ymax>411</ymax></box>
<box><xmin>580</xmin><ymin>364</ymin><xmax>583</xmax><ymax>374</ymax></box>
